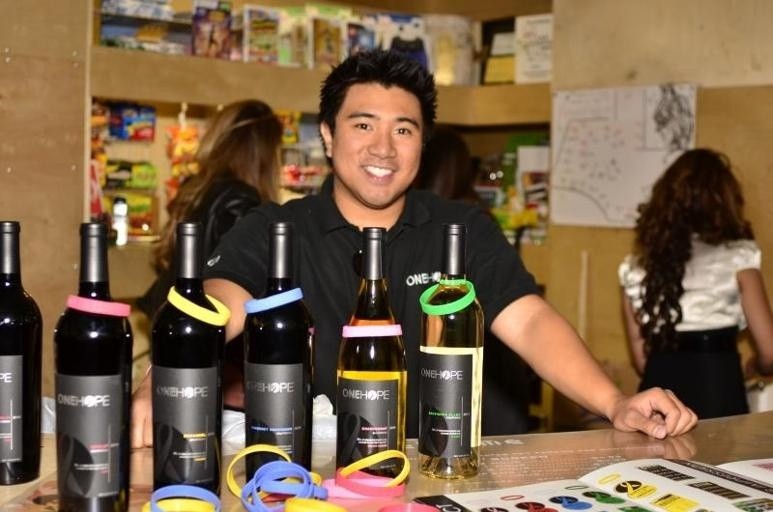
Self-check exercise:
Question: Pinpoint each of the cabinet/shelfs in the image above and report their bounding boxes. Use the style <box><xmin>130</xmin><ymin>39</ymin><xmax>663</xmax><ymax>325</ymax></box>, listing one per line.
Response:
<box><xmin>92</xmin><ymin>0</ymin><xmax>554</xmax><ymax>302</ymax></box>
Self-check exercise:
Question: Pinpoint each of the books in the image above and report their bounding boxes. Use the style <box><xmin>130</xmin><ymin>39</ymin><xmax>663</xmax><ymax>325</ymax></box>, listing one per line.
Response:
<box><xmin>413</xmin><ymin>456</ymin><xmax>773</xmax><ymax>512</ymax></box>
<box><xmin>99</xmin><ymin>2</ymin><xmax>553</xmax><ymax>86</ymax></box>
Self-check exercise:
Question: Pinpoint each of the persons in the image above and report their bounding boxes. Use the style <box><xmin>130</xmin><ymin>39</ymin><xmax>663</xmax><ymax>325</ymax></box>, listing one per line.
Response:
<box><xmin>146</xmin><ymin>101</ymin><xmax>287</xmax><ymax>274</ymax></box>
<box><xmin>407</xmin><ymin>125</ymin><xmax>488</xmax><ymax>207</ymax></box>
<box><xmin>123</xmin><ymin>49</ymin><xmax>701</xmax><ymax>449</ymax></box>
<box><xmin>615</xmin><ymin>148</ymin><xmax>773</xmax><ymax>419</ymax></box>
<box><xmin>484</xmin><ymin>431</ymin><xmax>696</xmax><ymax>487</ymax></box>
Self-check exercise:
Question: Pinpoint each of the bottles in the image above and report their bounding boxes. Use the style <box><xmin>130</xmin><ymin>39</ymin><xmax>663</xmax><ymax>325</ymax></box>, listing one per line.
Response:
<box><xmin>52</xmin><ymin>220</ymin><xmax>133</xmax><ymax>511</ymax></box>
<box><xmin>151</xmin><ymin>221</ymin><xmax>225</xmax><ymax>500</ymax></box>
<box><xmin>0</xmin><ymin>220</ymin><xmax>42</xmax><ymax>485</ymax></box>
<box><xmin>418</xmin><ymin>221</ymin><xmax>484</xmax><ymax>481</ymax></box>
<box><xmin>337</xmin><ymin>227</ymin><xmax>409</xmax><ymax>481</ymax></box>
<box><xmin>242</xmin><ymin>221</ymin><xmax>316</xmax><ymax>492</ymax></box>
